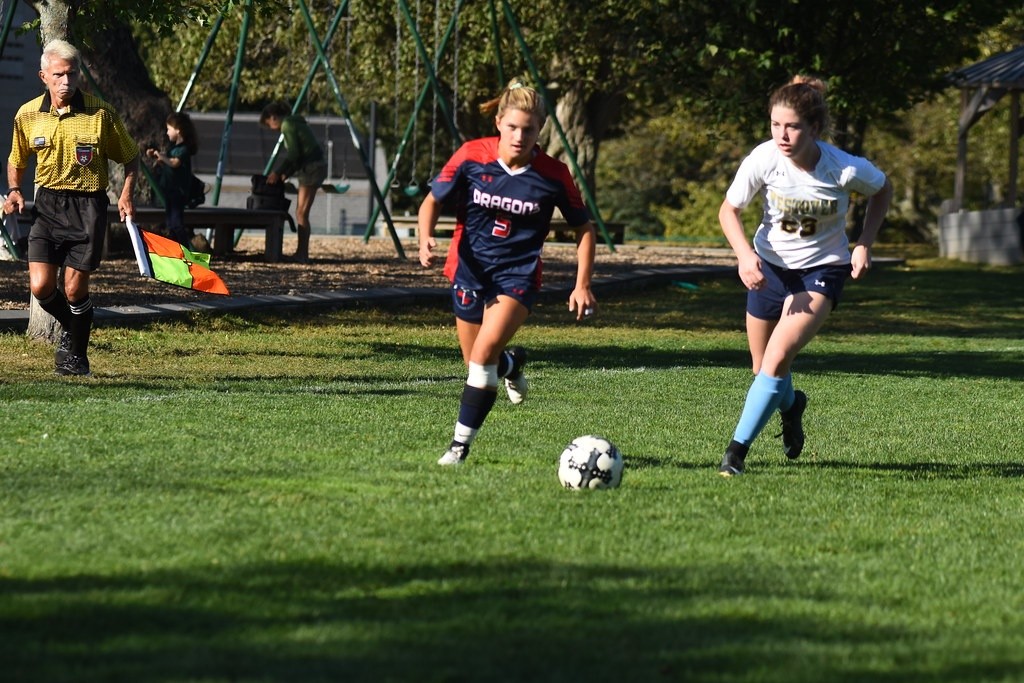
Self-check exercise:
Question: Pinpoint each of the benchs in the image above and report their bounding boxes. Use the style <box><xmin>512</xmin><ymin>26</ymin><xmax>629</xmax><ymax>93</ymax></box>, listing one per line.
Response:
<box><xmin>0</xmin><ymin>203</ymin><xmax>288</xmax><ymax>264</ymax></box>
<box><xmin>545</xmin><ymin>217</ymin><xmax>629</xmax><ymax>246</ymax></box>
<box><xmin>380</xmin><ymin>214</ymin><xmax>457</xmax><ymax>239</ymax></box>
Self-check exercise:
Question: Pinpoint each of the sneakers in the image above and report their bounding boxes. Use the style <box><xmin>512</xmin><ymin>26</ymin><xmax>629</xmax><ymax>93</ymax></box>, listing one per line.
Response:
<box><xmin>719</xmin><ymin>440</ymin><xmax>750</xmax><ymax>478</ymax></box>
<box><xmin>54</xmin><ymin>355</ymin><xmax>90</xmax><ymax>377</ymax></box>
<box><xmin>778</xmin><ymin>388</ymin><xmax>806</xmax><ymax>460</ymax></box>
<box><xmin>53</xmin><ymin>330</ymin><xmax>75</xmax><ymax>368</ymax></box>
<box><xmin>505</xmin><ymin>344</ymin><xmax>528</xmax><ymax>404</ymax></box>
<box><xmin>437</xmin><ymin>451</ymin><xmax>465</xmax><ymax>467</ymax></box>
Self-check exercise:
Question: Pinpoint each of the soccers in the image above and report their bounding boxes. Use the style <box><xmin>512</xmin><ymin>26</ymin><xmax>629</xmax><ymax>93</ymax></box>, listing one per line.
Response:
<box><xmin>558</xmin><ymin>435</ymin><xmax>625</xmax><ymax>490</ymax></box>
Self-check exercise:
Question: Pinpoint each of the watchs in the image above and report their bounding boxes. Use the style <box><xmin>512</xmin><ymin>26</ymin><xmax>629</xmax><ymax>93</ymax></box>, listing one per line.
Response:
<box><xmin>6</xmin><ymin>187</ymin><xmax>24</xmax><ymax>197</ymax></box>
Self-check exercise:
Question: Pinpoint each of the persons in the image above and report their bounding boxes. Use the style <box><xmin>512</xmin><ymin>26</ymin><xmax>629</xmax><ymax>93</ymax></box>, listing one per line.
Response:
<box><xmin>257</xmin><ymin>101</ymin><xmax>326</xmax><ymax>266</ymax></box>
<box><xmin>146</xmin><ymin>111</ymin><xmax>199</xmax><ymax>250</ymax></box>
<box><xmin>717</xmin><ymin>75</ymin><xmax>895</xmax><ymax>477</ymax></box>
<box><xmin>415</xmin><ymin>74</ymin><xmax>601</xmax><ymax>469</ymax></box>
<box><xmin>4</xmin><ymin>38</ymin><xmax>144</xmax><ymax>375</ymax></box>
